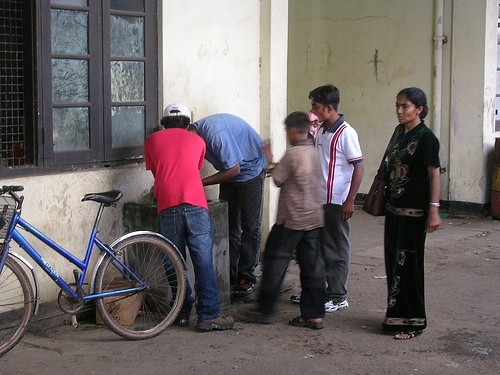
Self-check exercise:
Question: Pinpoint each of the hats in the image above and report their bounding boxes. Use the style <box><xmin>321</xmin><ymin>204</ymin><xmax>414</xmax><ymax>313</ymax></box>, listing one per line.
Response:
<box><xmin>162</xmin><ymin>103</ymin><xmax>191</xmax><ymax>121</ymax></box>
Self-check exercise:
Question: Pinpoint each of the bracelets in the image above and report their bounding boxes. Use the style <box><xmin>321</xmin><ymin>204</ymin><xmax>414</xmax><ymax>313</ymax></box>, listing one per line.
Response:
<box><xmin>430</xmin><ymin>203</ymin><xmax>441</xmax><ymax>208</ymax></box>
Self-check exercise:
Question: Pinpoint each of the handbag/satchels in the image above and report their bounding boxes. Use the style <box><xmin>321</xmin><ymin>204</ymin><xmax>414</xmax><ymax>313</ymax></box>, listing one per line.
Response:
<box><xmin>362</xmin><ymin>171</ymin><xmax>392</xmax><ymax>217</ymax></box>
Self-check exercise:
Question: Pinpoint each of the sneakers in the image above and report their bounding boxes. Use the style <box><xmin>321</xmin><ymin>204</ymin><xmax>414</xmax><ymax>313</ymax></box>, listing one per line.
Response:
<box><xmin>290</xmin><ymin>291</ymin><xmax>302</xmax><ymax>304</ymax></box>
<box><xmin>324</xmin><ymin>298</ymin><xmax>349</xmax><ymax>312</ymax></box>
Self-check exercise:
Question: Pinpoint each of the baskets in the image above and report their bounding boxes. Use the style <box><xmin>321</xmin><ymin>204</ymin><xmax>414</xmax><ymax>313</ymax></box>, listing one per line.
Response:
<box><xmin>0</xmin><ymin>195</ymin><xmax>17</xmax><ymax>243</ymax></box>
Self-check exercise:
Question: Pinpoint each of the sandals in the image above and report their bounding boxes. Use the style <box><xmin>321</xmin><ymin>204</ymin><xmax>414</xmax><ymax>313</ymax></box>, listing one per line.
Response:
<box><xmin>288</xmin><ymin>315</ymin><xmax>325</xmax><ymax>329</ymax></box>
<box><xmin>393</xmin><ymin>330</ymin><xmax>424</xmax><ymax>340</ymax></box>
<box><xmin>240</xmin><ymin>307</ymin><xmax>274</xmax><ymax>323</ymax></box>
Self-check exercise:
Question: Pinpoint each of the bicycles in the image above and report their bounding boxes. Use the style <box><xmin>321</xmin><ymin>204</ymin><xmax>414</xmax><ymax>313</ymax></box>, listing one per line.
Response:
<box><xmin>0</xmin><ymin>184</ymin><xmax>189</xmax><ymax>357</ymax></box>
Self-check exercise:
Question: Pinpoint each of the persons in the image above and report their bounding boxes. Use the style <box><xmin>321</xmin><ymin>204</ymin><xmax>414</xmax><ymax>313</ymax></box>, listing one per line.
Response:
<box><xmin>383</xmin><ymin>88</ymin><xmax>442</xmax><ymax>339</ymax></box>
<box><xmin>290</xmin><ymin>86</ymin><xmax>364</xmax><ymax>311</ymax></box>
<box><xmin>145</xmin><ymin>103</ymin><xmax>234</xmax><ymax>332</ymax></box>
<box><xmin>234</xmin><ymin>111</ymin><xmax>326</xmax><ymax>332</ymax></box>
<box><xmin>187</xmin><ymin>114</ymin><xmax>269</xmax><ymax>303</ymax></box>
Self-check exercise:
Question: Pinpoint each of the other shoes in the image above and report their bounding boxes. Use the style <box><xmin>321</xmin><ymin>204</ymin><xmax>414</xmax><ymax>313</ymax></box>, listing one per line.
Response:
<box><xmin>234</xmin><ymin>278</ymin><xmax>255</xmax><ymax>298</ymax></box>
<box><xmin>195</xmin><ymin>314</ymin><xmax>234</xmax><ymax>331</ymax></box>
<box><xmin>173</xmin><ymin>317</ymin><xmax>190</xmax><ymax>326</ymax></box>
